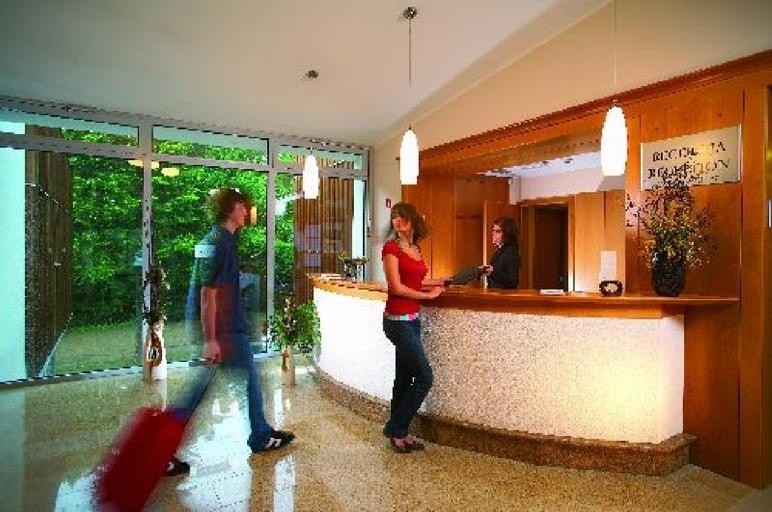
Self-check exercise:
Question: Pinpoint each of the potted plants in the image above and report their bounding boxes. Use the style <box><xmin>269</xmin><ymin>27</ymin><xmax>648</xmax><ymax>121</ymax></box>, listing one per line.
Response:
<box><xmin>264</xmin><ymin>297</ymin><xmax>323</xmax><ymax>388</ymax></box>
<box><xmin>137</xmin><ymin>265</ymin><xmax>169</xmax><ymax>379</ymax></box>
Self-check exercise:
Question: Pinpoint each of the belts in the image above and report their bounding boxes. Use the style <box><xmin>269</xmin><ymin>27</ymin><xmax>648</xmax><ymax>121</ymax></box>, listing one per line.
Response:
<box><xmin>383</xmin><ymin>311</ymin><xmax>419</xmax><ymax>321</ymax></box>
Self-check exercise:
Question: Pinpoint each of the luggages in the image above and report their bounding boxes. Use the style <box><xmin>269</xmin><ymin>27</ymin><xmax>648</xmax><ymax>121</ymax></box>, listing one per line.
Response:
<box><xmin>97</xmin><ymin>365</ymin><xmax>217</xmax><ymax>512</ymax></box>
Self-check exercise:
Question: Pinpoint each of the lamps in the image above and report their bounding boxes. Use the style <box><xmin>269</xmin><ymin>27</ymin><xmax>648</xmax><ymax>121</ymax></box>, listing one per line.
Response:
<box><xmin>398</xmin><ymin>4</ymin><xmax>421</xmax><ymax>187</ymax></box>
<box><xmin>599</xmin><ymin>0</ymin><xmax>629</xmax><ymax>177</ymax></box>
<box><xmin>302</xmin><ymin>135</ymin><xmax>320</xmax><ymax>200</ymax></box>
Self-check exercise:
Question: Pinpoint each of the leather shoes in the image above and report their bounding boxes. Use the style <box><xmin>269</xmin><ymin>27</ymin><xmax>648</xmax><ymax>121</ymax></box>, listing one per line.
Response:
<box><xmin>383</xmin><ymin>430</ymin><xmax>424</xmax><ymax>451</ymax></box>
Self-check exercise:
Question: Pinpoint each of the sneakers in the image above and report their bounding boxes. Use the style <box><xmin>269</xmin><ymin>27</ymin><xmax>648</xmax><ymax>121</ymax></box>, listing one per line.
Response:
<box><xmin>163</xmin><ymin>457</ymin><xmax>190</xmax><ymax>477</ymax></box>
<box><xmin>261</xmin><ymin>431</ymin><xmax>293</xmax><ymax>451</ymax></box>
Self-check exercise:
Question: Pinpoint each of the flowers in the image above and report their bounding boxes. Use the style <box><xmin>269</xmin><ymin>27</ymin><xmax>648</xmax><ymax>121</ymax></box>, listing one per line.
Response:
<box><xmin>619</xmin><ymin>163</ymin><xmax>720</xmax><ymax>269</ymax></box>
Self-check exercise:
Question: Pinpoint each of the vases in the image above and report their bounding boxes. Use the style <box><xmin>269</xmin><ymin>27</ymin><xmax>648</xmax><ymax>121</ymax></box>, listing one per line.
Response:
<box><xmin>650</xmin><ymin>261</ymin><xmax>686</xmax><ymax>296</ymax></box>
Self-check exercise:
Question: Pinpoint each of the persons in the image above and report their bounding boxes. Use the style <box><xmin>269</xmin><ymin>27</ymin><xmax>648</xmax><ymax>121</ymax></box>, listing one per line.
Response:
<box><xmin>380</xmin><ymin>201</ymin><xmax>455</xmax><ymax>455</ymax></box>
<box><xmin>475</xmin><ymin>216</ymin><xmax>521</xmax><ymax>289</ymax></box>
<box><xmin>145</xmin><ymin>187</ymin><xmax>295</xmax><ymax>479</ymax></box>
